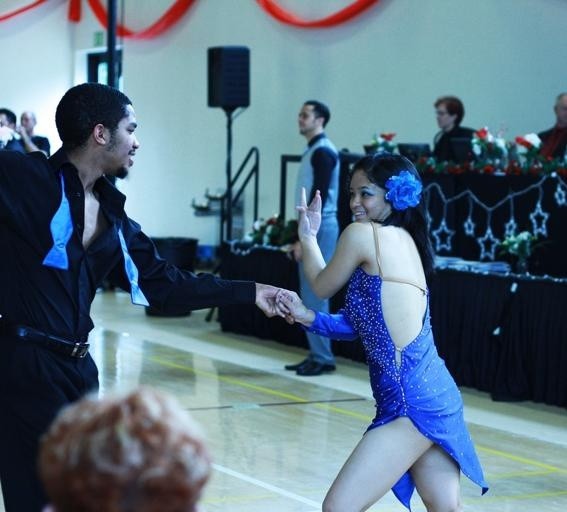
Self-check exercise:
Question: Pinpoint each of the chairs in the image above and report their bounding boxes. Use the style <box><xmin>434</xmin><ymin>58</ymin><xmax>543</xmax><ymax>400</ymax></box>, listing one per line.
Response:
<box><xmin>398</xmin><ymin>141</ymin><xmax>433</xmax><ymax>160</ymax></box>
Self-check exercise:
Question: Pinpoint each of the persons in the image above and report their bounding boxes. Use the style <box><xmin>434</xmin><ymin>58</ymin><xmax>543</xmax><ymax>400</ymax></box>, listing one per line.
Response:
<box><xmin>16</xmin><ymin>111</ymin><xmax>50</xmax><ymax>159</ymax></box>
<box><xmin>268</xmin><ymin>153</ymin><xmax>491</xmax><ymax>512</ymax></box>
<box><xmin>537</xmin><ymin>90</ymin><xmax>567</xmax><ymax>164</ymax></box>
<box><xmin>38</xmin><ymin>377</ymin><xmax>214</xmax><ymax>511</ymax></box>
<box><xmin>427</xmin><ymin>97</ymin><xmax>473</xmax><ymax>160</ymax></box>
<box><xmin>0</xmin><ymin>82</ymin><xmax>303</xmax><ymax>510</ymax></box>
<box><xmin>0</xmin><ymin>109</ymin><xmax>26</xmax><ymax>156</ymax></box>
<box><xmin>284</xmin><ymin>102</ymin><xmax>339</xmax><ymax>375</ymax></box>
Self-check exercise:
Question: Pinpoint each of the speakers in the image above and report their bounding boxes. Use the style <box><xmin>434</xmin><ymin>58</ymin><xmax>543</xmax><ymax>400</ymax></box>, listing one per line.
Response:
<box><xmin>208</xmin><ymin>46</ymin><xmax>249</xmax><ymax>108</ymax></box>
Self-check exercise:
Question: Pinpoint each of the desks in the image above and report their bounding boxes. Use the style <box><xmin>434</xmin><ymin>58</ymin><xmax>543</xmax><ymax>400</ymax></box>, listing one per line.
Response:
<box><xmin>212</xmin><ymin>239</ymin><xmax>567</xmax><ymax>411</ymax></box>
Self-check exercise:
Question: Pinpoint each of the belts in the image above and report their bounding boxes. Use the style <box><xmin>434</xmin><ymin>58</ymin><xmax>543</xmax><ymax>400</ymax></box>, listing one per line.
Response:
<box><xmin>4</xmin><ymin>323</ymin><xmax>90</xmax><ymax>358</ymax></box>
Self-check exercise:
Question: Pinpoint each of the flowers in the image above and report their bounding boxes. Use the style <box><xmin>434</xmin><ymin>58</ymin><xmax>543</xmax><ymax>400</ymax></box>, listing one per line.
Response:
<box><xmin>385</xmin><ymin>168</ymin><xmax>424</xmax><ymax>211</ymax></box>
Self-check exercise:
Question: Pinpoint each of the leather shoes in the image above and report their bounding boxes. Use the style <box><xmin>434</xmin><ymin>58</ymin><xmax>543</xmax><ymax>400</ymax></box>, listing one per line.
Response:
<box><xmin>285</xmin><ymin>360</ymin><xmax>336</xmax><ymax>375</ymax></box>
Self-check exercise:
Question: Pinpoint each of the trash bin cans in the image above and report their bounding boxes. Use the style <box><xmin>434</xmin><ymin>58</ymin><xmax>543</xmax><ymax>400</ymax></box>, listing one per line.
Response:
<box><xmin>145</xmin><ymin>237</ymin><xmax>198</xmax><ymax>316</ymax></box>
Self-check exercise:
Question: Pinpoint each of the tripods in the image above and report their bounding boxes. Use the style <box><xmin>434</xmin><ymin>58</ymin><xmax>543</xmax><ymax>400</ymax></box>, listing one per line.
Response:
<box><xmin>204</xmin><ymin>109</ymin><xmax>231</xmax><ymax>322</ymax></box>
